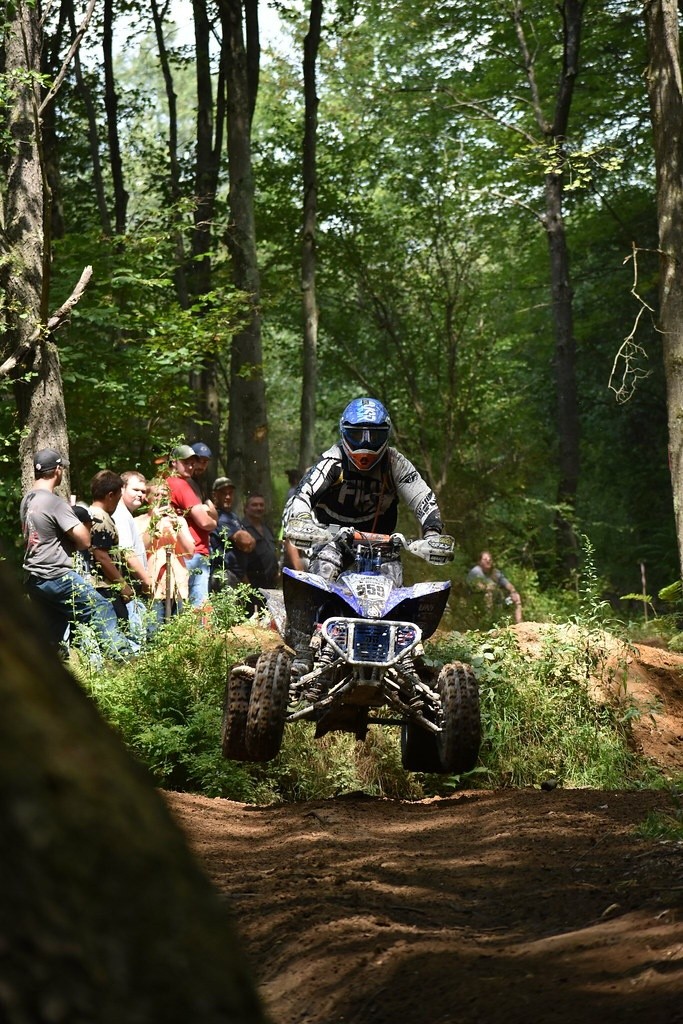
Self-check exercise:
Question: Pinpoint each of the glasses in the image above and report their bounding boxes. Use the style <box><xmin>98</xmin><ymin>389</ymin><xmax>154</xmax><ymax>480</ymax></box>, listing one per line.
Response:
<box><xmin>339</xmin><ymin>419</ymin><xmax>390</xmax><ymax>454</ymax></box>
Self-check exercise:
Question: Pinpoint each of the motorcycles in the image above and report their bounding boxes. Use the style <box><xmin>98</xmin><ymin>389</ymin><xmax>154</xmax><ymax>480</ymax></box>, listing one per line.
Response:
<box><xmin>217</xmin><ymin>518</ymin><xmax>483</xmax><ymax>776</ymax></box>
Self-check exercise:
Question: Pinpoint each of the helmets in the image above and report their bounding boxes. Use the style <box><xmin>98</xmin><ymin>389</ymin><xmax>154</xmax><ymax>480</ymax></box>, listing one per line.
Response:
<box><xmin>339</xmin><ymin>398</ymin><xmax>391</xmax><ymax>471</ymax></box>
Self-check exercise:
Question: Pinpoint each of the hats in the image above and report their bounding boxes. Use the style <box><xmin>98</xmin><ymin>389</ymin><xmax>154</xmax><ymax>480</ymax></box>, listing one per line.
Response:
<box><xmin>212</xmin><ymin>478</ymin><xmax>236</xmax><ymax>493</ymax></box>
<box><xmin>171</xmin><ymin>444</ymin><xmax>198</xmax><ymax>462</ymax></box>
<box><xmin>35</xmin><ymin>447</ymin><xmax>70</xmax><ymax>473</ymax></box>
<box><xmin>193</xmin><ymin>443</ymin><xmax>214</xmax><ymax>461</ymax></box>
<box><xmin>69</xmin><ymin>505</ymin><xmax>91</xmax><ymax>524</ymax></box>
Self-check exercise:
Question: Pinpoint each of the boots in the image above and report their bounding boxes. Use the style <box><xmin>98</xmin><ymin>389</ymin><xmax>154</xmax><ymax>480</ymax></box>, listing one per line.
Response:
<box><xmin>284</xmin><ymin>597</ymin><xmax>315</xmax><ymax>675</ymax></box>
<box><xmin>395</xmin><ymin>649</ymin><xmax>423</xmax><ymax>684</ymax></box>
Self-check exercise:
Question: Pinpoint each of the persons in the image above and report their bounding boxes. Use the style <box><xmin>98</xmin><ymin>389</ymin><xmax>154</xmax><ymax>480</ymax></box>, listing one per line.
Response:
<box><xmin>65</xmin><ymin>441</ymin><xmax>279</xmax><ymax>677</ymax></box>
<box><xmin>279</xmin><ymin>396</ymin><xmax>444</xmax><ymax>588</ymax></box>
<box><xmin>467</xmin><ymin>550</ymin><xmax>527</xmax><ymax>624</ymax></box>
<box><xmin>19</xmin><ymin>446</ymin><xmax>137</xmax><ymax>666</ymax></box>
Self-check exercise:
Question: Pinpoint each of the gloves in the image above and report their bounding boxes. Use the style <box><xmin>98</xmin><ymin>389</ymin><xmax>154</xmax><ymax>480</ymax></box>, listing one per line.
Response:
<box><xmin>424</xmin><ymin>531</ymin><xmax>450</xmax><ymax>566</ymax></box>
<box><xmin>290</xmin><ymin>513</ymin><xmax>319</xmax><ymax>548</ymax></box>
